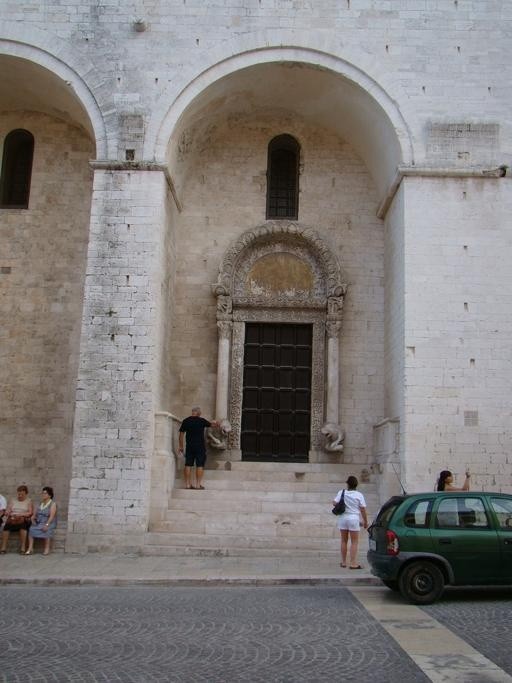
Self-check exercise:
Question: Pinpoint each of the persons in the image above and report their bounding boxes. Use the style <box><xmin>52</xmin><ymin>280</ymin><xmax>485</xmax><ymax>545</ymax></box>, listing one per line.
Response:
<box><xmin>25</xmin><ymin>487</ymin><xmax>56</xmax><ymax>555</ymax></box>
<box><xmin>0</xmin><ymin>485</ymin><xmax>32</xmax><ymax>555</ymax></box>
<box><xmin>0</xmin><ymin>493</ymin><xmax>7</xmax><ymax>518</ymax></box>
<box><xmin>332</xmin><ymin>476</ymin><xmax>368</xmax><ymax>568</ymax></box>
<box><xmin>178</xmin><ymin>406</ymin><xmax>221</xmax><ymax>489</ymax></box>
<box><xmin>437</xmin><ymin>468</ymin><xmax>472</xmax><ymax>491</ymax></box>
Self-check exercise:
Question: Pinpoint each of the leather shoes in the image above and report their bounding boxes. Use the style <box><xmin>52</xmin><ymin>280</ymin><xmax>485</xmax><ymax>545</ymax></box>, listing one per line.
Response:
<box><xmin>43</xmin><ymin>550</ymin><xmax>48</xmax><ymax>554</ymax></box>
<box><xmin>25</xmin><ymin>550</ymin><xmax>33</xmax><ymax>554</ymax></box>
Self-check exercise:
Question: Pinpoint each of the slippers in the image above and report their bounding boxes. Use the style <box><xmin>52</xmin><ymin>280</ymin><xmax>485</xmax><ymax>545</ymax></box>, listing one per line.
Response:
<box><xmin>186</xmin><ymin>484</ymin><xmax>194</xmax><ymax>488</ymax></box>
<box><xmin>340</xmin><ymin>562</ymin><xmax>346</xmax><ymax>567</ymax></box>
<box><xmin>195</xmin><ymin>484</ymin><xmax>205</xmax><ymax>489</ymax></box>
<box><xmin>0</xmin><ymin>550</ymin><xmax>6</xmax><ymax>553</ymax></box>
<box><xmin>350</xmin><ymin>564</ymin><xmax>362</xmax><ymax>569</ymax></box>
<box><xmin>19</xmin><ymin>549</ymin><xmax>26</xmax><ymax>554</ymax></box>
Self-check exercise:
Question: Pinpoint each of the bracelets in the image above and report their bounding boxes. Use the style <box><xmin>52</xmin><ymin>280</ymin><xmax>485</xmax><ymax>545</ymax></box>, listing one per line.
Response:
<box><xmin>45</xmin><ymin>524</ymin><xmax>49</xmax><ymax>526</ymax></box>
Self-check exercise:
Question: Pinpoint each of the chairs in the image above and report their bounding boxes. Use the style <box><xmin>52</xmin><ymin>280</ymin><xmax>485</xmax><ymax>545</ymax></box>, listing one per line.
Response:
<box><xmin>424</xmin><ymin>512</ymin><xmax>440</xmax><ymax>526</ymax></box>
<box><xmin>458</xmin><ymin>509</ymin><xmax>476</xmax><ymax>528</ymax></box>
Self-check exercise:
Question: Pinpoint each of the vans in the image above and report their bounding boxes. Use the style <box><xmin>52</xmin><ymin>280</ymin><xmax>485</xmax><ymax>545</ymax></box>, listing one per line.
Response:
<box><xmin>366</xmin><ymin>489</ymin><xmax>511</xmax><ymax>606</ymax></box>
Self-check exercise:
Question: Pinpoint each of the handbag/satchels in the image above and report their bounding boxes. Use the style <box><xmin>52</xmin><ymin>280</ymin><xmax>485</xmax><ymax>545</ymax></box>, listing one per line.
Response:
<box><xmin>332</xmin><ymin>488</ymin><xmax>346</xmax><ymax>514</ymax></box>
<box><xmin>7</xmin><ymin>515</ymin><xmax>25</xmax><ymax>525</ymax></box>
